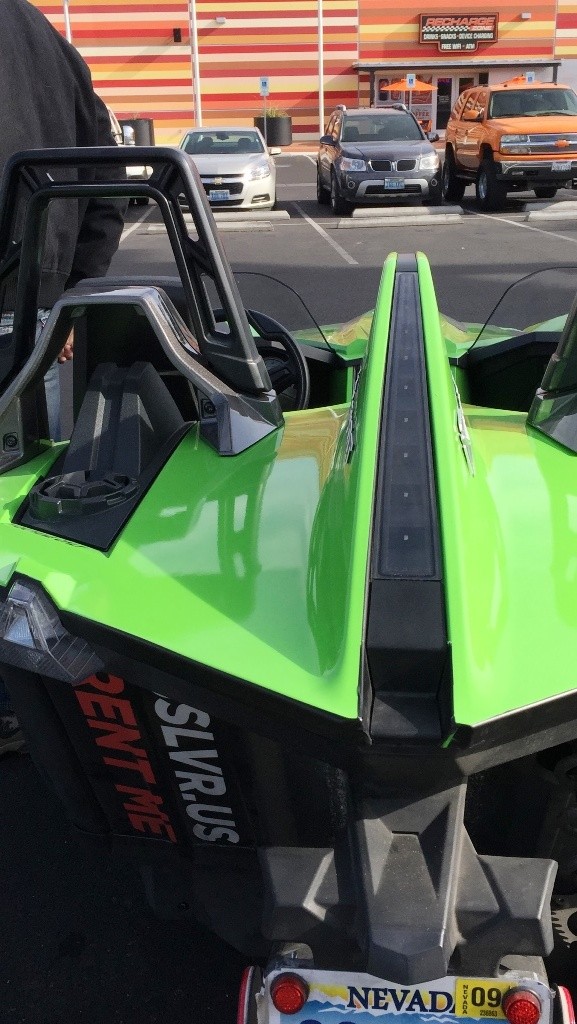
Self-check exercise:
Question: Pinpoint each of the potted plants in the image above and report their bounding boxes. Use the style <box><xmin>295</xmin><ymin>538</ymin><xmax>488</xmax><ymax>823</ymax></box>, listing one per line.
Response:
<box><xmin>254</xmin><ymin>107</ymin><xmax>292</xmax><ymax>147</ymax></box>
<box><xmin>117</xmin><ymin>114</ymin><xmax>155</xmax><ymax>146</ymax></box>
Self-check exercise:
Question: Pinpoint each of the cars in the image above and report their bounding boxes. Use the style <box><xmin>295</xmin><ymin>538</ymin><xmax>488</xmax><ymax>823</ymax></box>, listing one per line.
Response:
<box><xmin>0</xmin><ymin>144</ymin><xmax>577</xmax><ymax>1024</ymax></box>
<box><xmin>168</xmin><ymin>125</ymin><xmax>282</xmax><ymax>213</ymax></box>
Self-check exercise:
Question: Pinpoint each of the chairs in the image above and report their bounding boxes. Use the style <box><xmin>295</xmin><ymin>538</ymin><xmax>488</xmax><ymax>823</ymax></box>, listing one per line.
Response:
<box><xmin>199</xmin><ymin>137</ymin><xmax>213</xmax><ymax>149</ymax></box>
<box><xmin>343</xmin><ymin>127</ymin><xmax>361</xmax><ymax>143</ymax></box>
<box><xmin>69</xmin><ymin>275</ymin><xmax>205</xmax><ymax>425</ymax></box>
<box><xmin>238</xmin><ymin>138</ymin><xmax>251</xmax><ymax>151</ymax></box>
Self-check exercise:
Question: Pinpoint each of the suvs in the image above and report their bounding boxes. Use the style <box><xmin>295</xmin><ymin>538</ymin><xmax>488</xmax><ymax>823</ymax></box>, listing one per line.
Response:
<box><xmin>315</xmin><ymin>103</ymin><xmax>444</xmax><ymax>216</ymax></box>
<box><xmin>440</xmin><ymin>75</ymin><xmax>577</xmax><ymax>211</ymax></box>
